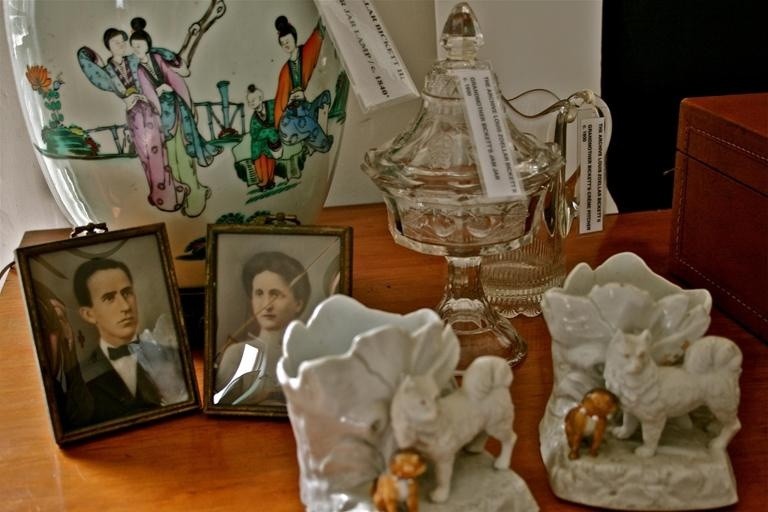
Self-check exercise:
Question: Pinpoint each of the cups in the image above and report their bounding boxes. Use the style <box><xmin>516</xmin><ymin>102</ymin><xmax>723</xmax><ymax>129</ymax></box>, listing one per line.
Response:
<box><xmin>471</xmin><ymin>159</ymin><xmax>562</xmax><ymax>329</ymax></box>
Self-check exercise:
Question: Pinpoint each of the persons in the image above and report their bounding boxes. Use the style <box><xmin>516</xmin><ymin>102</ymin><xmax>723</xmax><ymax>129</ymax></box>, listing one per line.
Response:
<box><xmin>57</xmin><ymin>255</ymin><xmax>185</xmax><ymax>431</ymax></box>
<box><xmin>213</xmin><ymin>250</ymin><xmax>313</xmax><ymax>409</ymax></box>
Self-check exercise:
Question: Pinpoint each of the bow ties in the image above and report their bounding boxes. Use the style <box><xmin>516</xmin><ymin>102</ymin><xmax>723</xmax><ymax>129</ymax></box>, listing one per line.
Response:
<box><xmin>107</xmin><ymin>341</ymin><xmax>139</xmax><ymax>360</ymax></box>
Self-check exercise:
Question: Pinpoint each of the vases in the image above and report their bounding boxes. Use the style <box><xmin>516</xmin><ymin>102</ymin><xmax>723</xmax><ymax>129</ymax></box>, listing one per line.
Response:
<box><xmin>2</xmin><ymin>0</ymin><xmax>353</xmax><ymax>293</ymax></box>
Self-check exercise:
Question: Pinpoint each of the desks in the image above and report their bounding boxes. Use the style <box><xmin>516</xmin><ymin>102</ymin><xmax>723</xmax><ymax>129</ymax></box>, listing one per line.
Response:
<box><xmin>0</xmin><ymin>201</ymin><xmax>768</xmax><ymax>511</ymax></box>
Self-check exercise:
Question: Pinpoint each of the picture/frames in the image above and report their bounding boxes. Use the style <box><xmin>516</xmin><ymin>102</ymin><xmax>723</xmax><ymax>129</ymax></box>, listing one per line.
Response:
<box><xmin>198</xmin><ymin>223</ymin><xmax>355</xmax><ymax>420</ymax></box>
<box><xmin>11</xmin><ymin>221</ymin><xmax>204</xmax><ymax>448</ymax></box>
